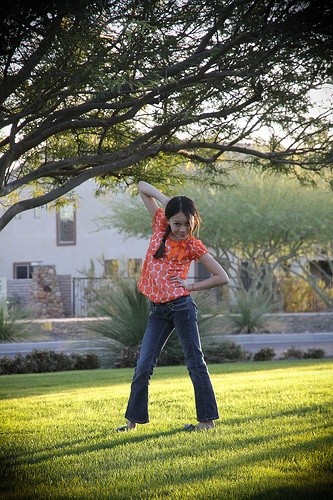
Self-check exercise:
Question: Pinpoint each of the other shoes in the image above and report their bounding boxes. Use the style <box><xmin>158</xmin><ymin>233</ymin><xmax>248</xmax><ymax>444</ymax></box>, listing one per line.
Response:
<box><xmin>186</xmin><ymin>424</ymin><xmax>214</xmax><ymax>432</ymax></box>
<box><xmin>116</xmin><ymin>425</ymin><xmax>132</xmax><ymax>432</ymax></box>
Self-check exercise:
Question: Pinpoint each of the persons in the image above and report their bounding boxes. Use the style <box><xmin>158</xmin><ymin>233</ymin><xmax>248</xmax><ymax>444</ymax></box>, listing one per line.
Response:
<box><xmin>114</xmin><ymin>181</ymin><xmax>229</xmax><ymax>432</ymax></box>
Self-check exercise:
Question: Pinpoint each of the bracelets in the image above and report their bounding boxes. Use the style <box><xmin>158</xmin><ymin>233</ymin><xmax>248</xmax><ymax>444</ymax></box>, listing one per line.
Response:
<box><xmin>191</xmin><ymin>283</ymin><xmax>194</xmax><ymax>292</ymax></box>
